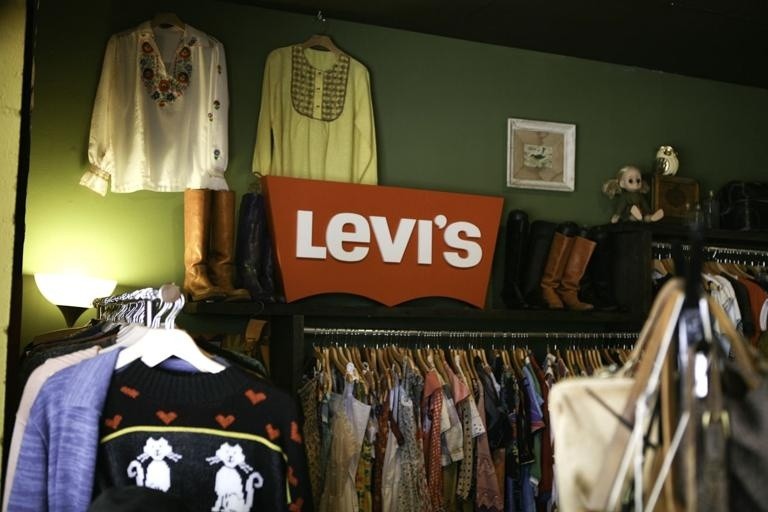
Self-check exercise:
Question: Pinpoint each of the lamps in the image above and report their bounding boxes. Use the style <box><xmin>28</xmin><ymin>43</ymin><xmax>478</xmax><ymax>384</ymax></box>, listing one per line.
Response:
<box><xmin>34</xmin><ymin>275</ymin><xmax>116</xmax><ymax>328</ymax></box>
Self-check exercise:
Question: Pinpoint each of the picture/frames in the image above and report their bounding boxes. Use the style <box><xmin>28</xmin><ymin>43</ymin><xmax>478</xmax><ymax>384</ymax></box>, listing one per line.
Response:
<box><xmin>652</xmin><ymin>175</ymin><xmax>700</xmax><ymax>222</ymax></box>
<box><xmin>506</xmin><ymin>118</ymin><xmax>576</xmax><ymax>192</ymax></box>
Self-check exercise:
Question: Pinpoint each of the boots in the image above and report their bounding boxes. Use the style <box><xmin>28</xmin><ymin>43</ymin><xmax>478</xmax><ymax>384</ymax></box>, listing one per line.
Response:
<box><xmin>501</xmin><ymin>207</ymin><xmax>629</xmax><ymax>314</ymax></box>
<box><xmin>180</xmin><ymin>186</ymin><xmax>283</xmax><ymax>305</ymax></box>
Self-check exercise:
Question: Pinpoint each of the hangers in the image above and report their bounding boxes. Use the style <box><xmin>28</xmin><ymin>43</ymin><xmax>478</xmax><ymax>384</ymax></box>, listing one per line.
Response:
<box><xmin>95</xmin><ymin>287</ymin><xmax>226</xmax><ymax>373</ymax></box>
<box><xmin>310</xmin><ymin>328</ymin><xmax>638</xmax><ymax>395</ymax></box>
<box><xmin>152</xmin><ymin>12</ymin><xmax>184</xmax><ymax>32</ymax></box>
<box><xmin>302</xmin><ymin>18</ymin><xmax>339</xmax><ymax>56</ymax></box>
<box><xmin>652</xmin><ymin>242</ymin><xmax>768</xmax><ymax>281</ymax></box>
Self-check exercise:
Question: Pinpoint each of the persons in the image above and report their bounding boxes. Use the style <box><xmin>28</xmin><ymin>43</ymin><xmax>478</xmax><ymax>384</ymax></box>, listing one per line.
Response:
<box><xmin>605</xmin><ymin>163</ymin><xmax>664</xmax><ymax>223</ymax></box>
